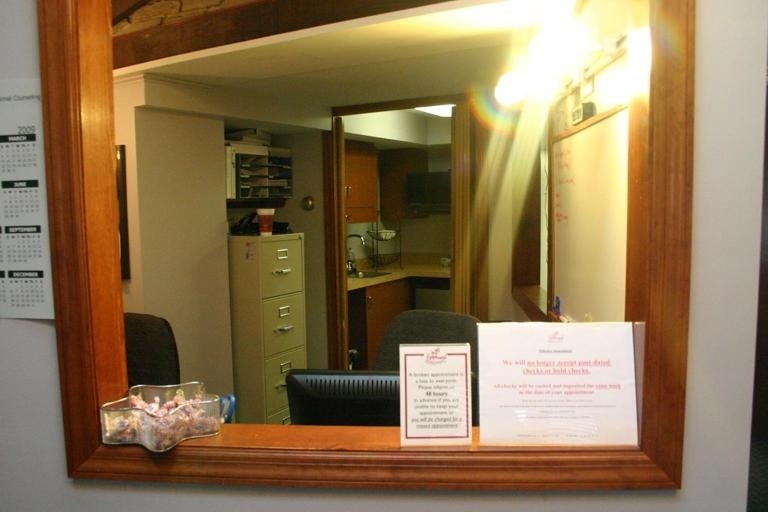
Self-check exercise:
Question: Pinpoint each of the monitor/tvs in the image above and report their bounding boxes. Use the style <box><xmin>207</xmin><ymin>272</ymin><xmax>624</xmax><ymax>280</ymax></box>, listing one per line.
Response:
<box><xmin>284</xmin><ymin>370</ymin><xmax>478</xmax><ymax>425</ymax></box>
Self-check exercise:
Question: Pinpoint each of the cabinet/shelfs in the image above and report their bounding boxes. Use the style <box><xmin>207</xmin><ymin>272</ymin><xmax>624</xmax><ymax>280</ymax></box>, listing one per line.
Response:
<box><xmin>225</xmin><ymin>143</ymin><xmax>295</xmax><ymax>201</ymax></box>
<box><xmin>365</xmin><ymin>279</ymin><xmax>413</xmax><ymax>365</ymax></box>
<box><xmin>343</xmin><ymin>139</ymin><xmax>378</xmax><ymax>224</ymax></box>
<box><xmin>230</xmin><ymin>234</ymin><xmax>308</xmax><ymax>425</ymax></box>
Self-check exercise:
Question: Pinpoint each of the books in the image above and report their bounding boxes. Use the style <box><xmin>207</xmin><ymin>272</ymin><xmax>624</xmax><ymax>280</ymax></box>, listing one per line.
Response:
<box><xmin>225</xmin><ymin>127</ymin><xmax>293</xmax><ymax>198</ymax></box>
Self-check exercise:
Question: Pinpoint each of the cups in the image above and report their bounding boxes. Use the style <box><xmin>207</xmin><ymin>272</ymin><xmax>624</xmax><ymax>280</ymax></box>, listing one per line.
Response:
<box><xmin>255</xmin><ymin>208</ymin><xmax>275</xmax><ymax>237</ymax></box>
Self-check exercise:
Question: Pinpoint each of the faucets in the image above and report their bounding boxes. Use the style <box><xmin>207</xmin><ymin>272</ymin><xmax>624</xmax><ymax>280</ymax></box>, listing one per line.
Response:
<box><xmin>346</xmin><ymin>234</ymin><xmax>366</xmax><ymax>246</ymax></box>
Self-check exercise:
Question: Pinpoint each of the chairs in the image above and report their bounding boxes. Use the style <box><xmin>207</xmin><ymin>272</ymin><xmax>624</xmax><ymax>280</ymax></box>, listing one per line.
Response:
<box><xmin>374</xmin><ymin>309</ymin><xmax>481</xmax><ymax>371</ymax></box>
<box><xmin>124</xmin><ymin>313</ymin><xmax>180</xmax><ymax>388</ymax></box>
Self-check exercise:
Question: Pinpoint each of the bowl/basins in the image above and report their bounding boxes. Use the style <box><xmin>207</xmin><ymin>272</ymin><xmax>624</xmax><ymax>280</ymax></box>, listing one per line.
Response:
<box><xmin>368</xmin><ymin>230</ymin><xmax>397</xmax><ymax>240</ymax></box>
<box><xmin>370</xmin><ymin>253</ymin><xmax>400</xmax><ymax>265</ymax></box>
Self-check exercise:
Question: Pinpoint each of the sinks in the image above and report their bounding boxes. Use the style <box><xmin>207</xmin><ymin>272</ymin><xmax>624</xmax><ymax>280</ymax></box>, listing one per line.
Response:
<box><xmin>347</xmin><ymin>271</ymin><xmax>391</xmax><ymax>279</ymax></box>
